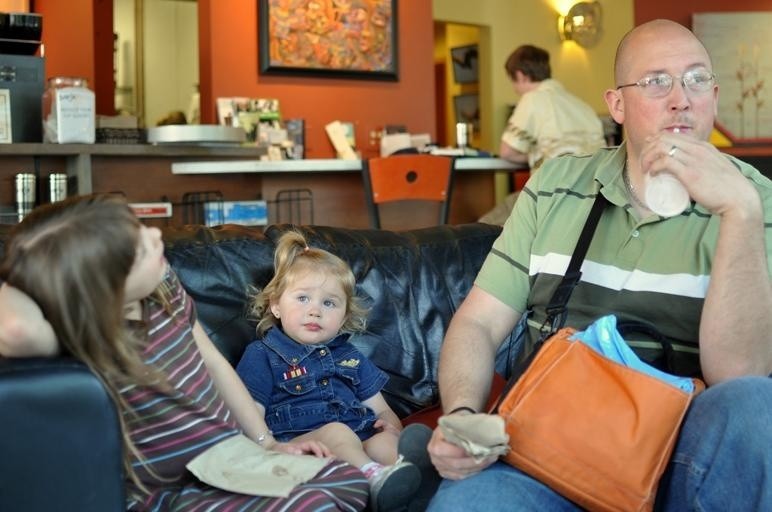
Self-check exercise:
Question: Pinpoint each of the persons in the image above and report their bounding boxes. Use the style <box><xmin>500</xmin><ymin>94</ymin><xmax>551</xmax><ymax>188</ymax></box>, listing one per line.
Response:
<box><xmin>494</xmin><ymin>44</ymin><xmax>609</xmax><ymax>177</ymax></box>
<box><xmin>234</xmin><ymin>228</ymin><xmax>435</xmax><ymax>510</ymax></box>
<box><xmin>425</xmin><ymin>16</ymin><xmax>772</xmax><ymax>512</ymax></box>
<box><xmin>0</xmin><ymin>188</ymin><xmax>371</xmax><ymax>512</ymax></box>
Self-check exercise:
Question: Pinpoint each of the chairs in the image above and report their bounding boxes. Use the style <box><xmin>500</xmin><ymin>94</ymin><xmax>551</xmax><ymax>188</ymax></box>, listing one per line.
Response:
<box><xmin>361</xmin><ymin>153</ymin><xmax>456</xmax><ymax>231</ymax></box>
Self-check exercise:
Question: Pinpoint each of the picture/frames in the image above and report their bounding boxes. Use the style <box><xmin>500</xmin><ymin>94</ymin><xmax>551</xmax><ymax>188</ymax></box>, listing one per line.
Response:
<box><xmin>257</xmin><ymin>0</ymin><xmax>400</xmax><ymax>82</ymax></box>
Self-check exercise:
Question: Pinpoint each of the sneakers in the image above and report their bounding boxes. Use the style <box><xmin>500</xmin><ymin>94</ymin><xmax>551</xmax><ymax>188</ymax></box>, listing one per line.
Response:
<box><xmin>399</xmin><ymin>424</ymin><xmax>441</xmax><ymax>499</ymax></box>
<box><xmin>369</xmin><ymin>455</ymin><xmax>421</xmax><ymax>512</ymax></box>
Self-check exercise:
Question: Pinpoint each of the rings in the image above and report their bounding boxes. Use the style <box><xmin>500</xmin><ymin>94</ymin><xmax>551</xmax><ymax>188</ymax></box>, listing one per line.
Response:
<box><xmin>666</xmin><ymin>144</ymin><xmax>680</xmax><ymax>160</ymax></box>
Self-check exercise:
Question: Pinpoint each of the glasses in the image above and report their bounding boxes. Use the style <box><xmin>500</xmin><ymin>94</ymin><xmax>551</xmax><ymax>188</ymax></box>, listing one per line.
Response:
<box><xmin>616</xmin><ymin>67</ymin><xmax>715</xmax><ymax>98</ymax></box>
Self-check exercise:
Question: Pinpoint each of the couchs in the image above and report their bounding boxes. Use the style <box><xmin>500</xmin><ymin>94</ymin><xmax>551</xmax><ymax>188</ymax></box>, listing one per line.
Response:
<box><xmin>0</xmin><ymin>221</ymin><xmax>504</xmax><ymax>512</ymax></box>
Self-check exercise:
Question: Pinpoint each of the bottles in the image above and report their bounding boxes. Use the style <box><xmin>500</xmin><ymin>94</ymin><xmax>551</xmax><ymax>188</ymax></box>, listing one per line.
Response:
<box><xmin>41</xmin><ymin>74</ymin><xmax>96</xmax><ymax>147</ymax></box>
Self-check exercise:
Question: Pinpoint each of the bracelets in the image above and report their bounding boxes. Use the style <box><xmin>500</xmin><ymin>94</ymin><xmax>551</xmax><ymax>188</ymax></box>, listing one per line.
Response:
<box><xmin>443</xmin><ymin>404</ymin><xmax>480</xmax><ymax>417</ymax></box>
<box><xmin>253</xmin><ymin>428</ymin><xmax>275</xmax><ymax>445</ymax></box>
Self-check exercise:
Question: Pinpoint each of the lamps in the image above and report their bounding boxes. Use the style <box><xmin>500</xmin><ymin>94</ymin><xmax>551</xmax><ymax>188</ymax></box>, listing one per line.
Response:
<box><xmin>557</xmin><ymin>0</ymin><xmax>606</xmax><ymax>49</ymax></box>
